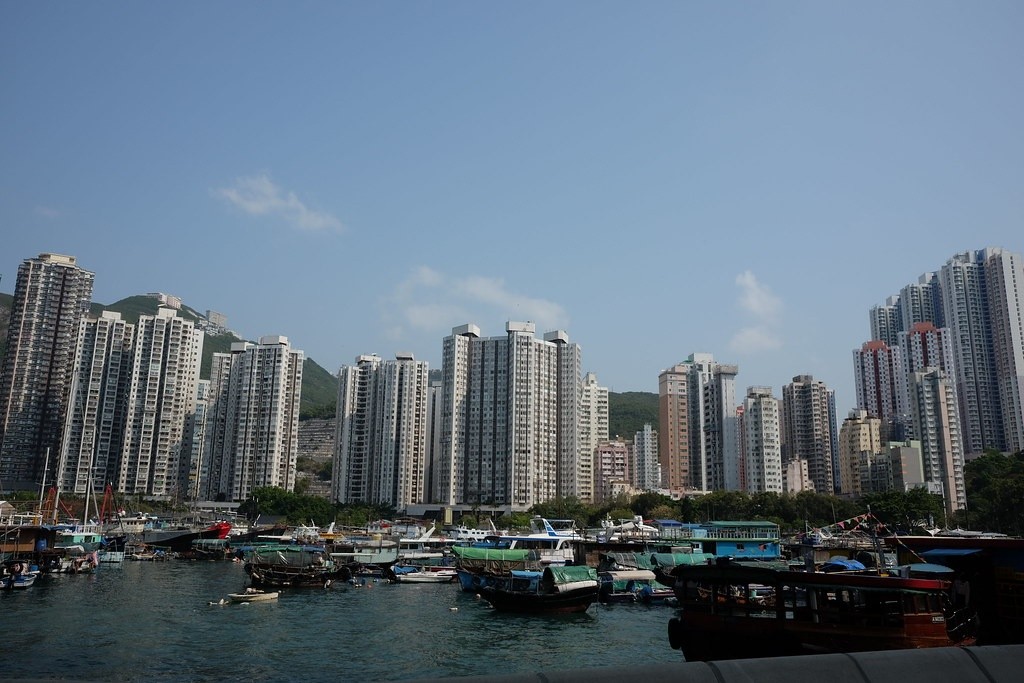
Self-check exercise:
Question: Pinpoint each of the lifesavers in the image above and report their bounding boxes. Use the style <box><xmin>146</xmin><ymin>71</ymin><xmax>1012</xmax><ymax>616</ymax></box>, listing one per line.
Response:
<box><xmin>472</xmin><ymin>576</ymin><xmax>481</xmax><ymax>585</ymax></box>
<box><xmin>8</xmin><ymin>562</ymin><xmax>23</xmax><ymax>574</ymax></box>
<box><xmin>486</xmin><ymin>576</ymin><xmax>496</xmax><ymax>586</ymax></box>
<box><xmin>297</xmin><ymin>574</ymin><xmax>304</xmax><ymax>581</ymax></box>
<box><xmin>282</xmin><ymin>574</ymin><xmax>288</xmax><ymax>581</ymax></box>
<box><xmin>498</xmin><ymin>579</ymin><xmax>506</xmax><ymax>587</ymax></box>
<box><xmin>668</xmin><ymin>618</ymin><xmax>683</xmax><ymax>651</ymax></box>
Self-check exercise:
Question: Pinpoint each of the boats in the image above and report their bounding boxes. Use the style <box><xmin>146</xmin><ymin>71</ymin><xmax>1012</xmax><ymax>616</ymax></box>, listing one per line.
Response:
<box><xmin>0</xmin><ymin>447</ymin><xmax>1024</xmax><ymax>662</ymax></box>
